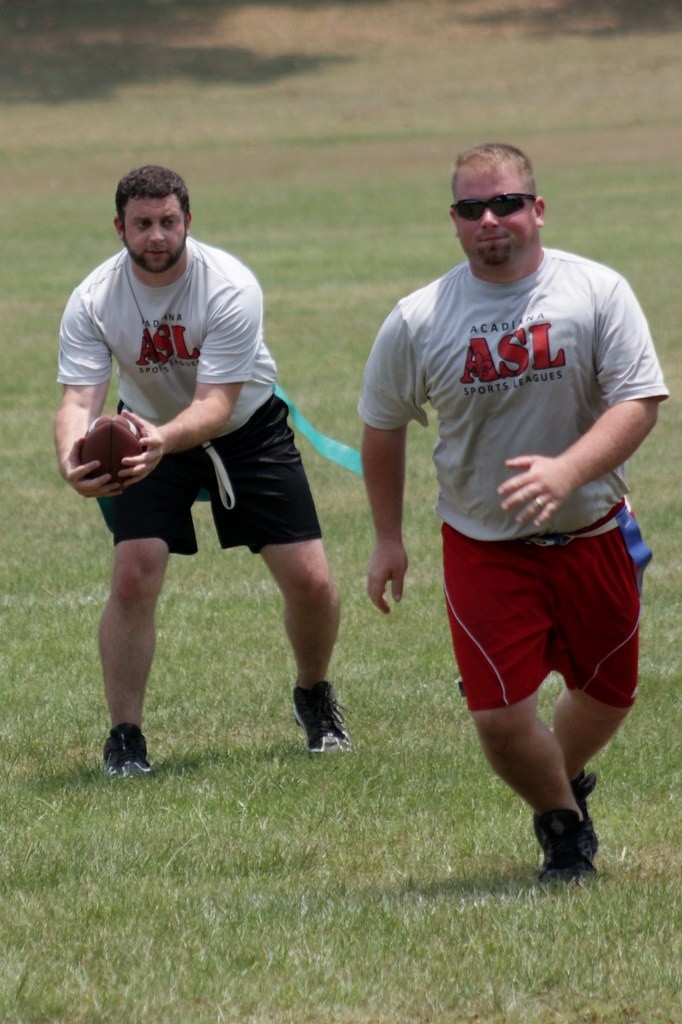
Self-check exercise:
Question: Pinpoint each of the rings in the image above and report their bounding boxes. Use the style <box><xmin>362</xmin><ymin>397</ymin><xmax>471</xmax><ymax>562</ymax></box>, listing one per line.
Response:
<box><xmin>535</xmin><ymin>498</ymin><xmax>543</xmax><ymax>506</ymax></box>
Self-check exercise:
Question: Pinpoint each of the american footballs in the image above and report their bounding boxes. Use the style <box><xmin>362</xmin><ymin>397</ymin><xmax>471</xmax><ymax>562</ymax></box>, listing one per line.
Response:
<box><xmin>80</xmin><ymin>412</ymin><xmax>143</xmax><ymax>496</ymax></box>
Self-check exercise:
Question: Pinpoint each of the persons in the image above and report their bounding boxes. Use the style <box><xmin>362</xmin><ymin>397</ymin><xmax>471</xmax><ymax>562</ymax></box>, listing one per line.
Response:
<box><xmin>357</xmin><ymin>144</ymin><xmax>671</xmax><ymax>881</ymax></box>
<box><xmin>55</xmin><ymin>165</ymin><xmax>350</xmax><ymax>779</ymax></box>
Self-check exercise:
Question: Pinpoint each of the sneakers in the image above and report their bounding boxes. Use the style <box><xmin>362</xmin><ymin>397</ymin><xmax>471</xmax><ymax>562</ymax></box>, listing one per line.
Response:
<box><xmin>103</xmin><ymin>724</ymin><xmax>152</xmax><ymax>780</ymax></box>
<box><xmin>571</xmin><ymin>770</ymin><xmax>594</xmax><ymax>817</ymax></box>
<box><xmin>292</xmin><ymin>680</ymin><xmax>351</xmax><ymax>752</ymax></box>
<box><xmin>531</xmin><ymin>808</ymin><xmax>600</xmax><ymax>886</ymax></box>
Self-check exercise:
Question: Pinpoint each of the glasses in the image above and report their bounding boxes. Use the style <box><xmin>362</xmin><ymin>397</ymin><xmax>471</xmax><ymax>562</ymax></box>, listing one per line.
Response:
<box><xmin>450</xmin><ymin>193</ymin><xmax>537</xmax><ymax>221</ymax></box>
